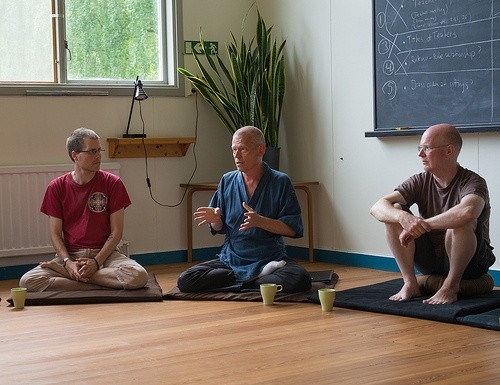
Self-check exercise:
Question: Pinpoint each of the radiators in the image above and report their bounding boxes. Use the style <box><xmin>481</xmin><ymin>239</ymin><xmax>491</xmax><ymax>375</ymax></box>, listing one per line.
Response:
<box><xmin>0</xmin><ymin>163</ymin><xmax>120</xmax><ymax>258</ymax></box>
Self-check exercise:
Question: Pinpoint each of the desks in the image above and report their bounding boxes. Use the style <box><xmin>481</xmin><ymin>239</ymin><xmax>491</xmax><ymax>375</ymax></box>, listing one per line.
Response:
<box><xmin>181</xmin><ymin>180</ymin><xmax>322</xmax><ymax>264</ymax></box>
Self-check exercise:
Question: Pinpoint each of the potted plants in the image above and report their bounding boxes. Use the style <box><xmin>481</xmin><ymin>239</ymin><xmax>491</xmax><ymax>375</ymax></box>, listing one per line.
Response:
<box><xmin>176</xmin><ymin>0</ymin><xmax>287</xmax><ymax>171</ymax></box>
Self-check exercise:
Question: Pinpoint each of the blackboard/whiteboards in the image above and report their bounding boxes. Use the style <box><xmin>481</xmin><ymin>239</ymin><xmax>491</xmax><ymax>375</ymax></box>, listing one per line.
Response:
<box><xmin>365</xmin><ymin>0</ymin><xmax>500</xmax><ymax>137</ymax></box>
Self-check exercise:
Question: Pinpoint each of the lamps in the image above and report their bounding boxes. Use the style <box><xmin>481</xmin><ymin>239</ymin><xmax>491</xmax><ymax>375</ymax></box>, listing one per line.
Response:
<box><xmin>123</xmin><ymin>76</ymin><xmax>148</xmax><ymax>138</ymax></box>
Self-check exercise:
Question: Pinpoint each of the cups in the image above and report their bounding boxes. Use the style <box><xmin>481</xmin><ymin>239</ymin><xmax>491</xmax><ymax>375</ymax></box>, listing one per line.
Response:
<box><xmin>260</xmin><ymin>284</ymin><xmax>283</xmax><ymax>305</ymax></box>
<box><xmin>318</xmin><ymin>289</ymin><xmax>336</xmax><ymax>311</ymax></box>
<box><xmin>11</xmin><ymin>287</ymin><xmax>27</xmax><ymax>309</ymax></box>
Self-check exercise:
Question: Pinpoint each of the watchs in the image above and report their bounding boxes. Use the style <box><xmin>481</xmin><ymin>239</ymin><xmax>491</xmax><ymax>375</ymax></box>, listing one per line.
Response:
<box><xmin>63</xmin><ymin>256</ymin><xmax>73</xmax><ymax>267</ymax></box>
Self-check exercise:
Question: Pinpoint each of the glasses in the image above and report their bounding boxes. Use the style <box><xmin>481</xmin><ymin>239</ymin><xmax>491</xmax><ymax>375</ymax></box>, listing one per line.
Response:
<box><xmin>76</xmin><ymin>149</ymin><xmax>104</xmax><ymax>154</ymax></box>
<box><xmin>417</xmin><ymin>144</ymin><xmax>450</xmax><ymax>151</ymax></box>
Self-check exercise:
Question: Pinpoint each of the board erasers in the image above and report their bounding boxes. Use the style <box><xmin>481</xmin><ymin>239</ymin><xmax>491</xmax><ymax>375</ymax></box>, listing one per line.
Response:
<box><xmin>396</xmin><ymin>128</ymin><xmax>411</xmax><ymax>131</ymax></box>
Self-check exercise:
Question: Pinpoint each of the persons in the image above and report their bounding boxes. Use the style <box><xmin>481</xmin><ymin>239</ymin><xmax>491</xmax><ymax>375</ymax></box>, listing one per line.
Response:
<box><xmin>18</xmin><ymin>127</ymin><xmax>150</xmax><ymax>294</ymax></box>
<box><xmin>369</xmin><ymin>123</ymin><xmax>497</xmax><ymax>305</ymax></box>
<box><xmin>176</xmin><ymin>126</ymin><xmax>313</xmax><ymax>296</ymax></box>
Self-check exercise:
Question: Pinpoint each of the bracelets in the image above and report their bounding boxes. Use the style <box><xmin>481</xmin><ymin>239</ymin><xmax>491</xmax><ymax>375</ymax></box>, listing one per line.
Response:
<box><xmin>92</xmin><ymin>257</ymin><xmax>99</xmax><ymax>270</ymax></box>
